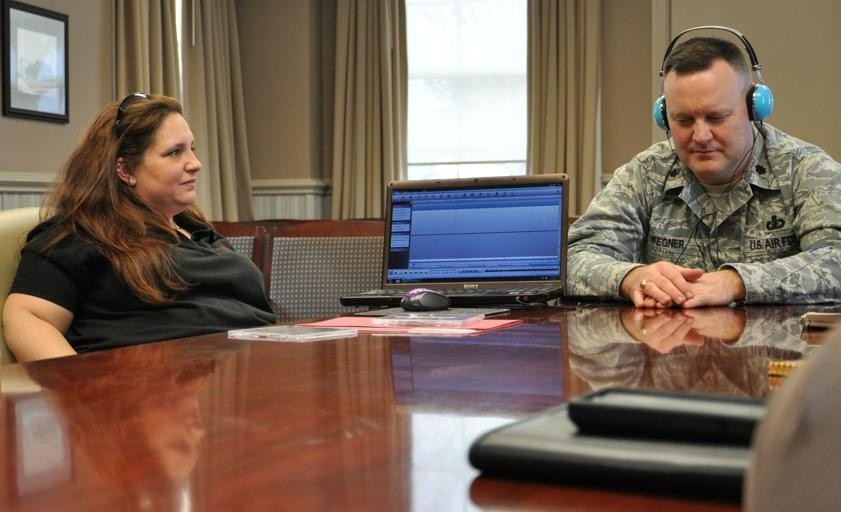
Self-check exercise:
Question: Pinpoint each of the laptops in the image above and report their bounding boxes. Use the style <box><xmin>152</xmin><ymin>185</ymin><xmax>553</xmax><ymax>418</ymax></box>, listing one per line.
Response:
<box><xmin>340</xmin><ymin>173</ymin><xmax>569</xmax><ymax>307</ymax></box>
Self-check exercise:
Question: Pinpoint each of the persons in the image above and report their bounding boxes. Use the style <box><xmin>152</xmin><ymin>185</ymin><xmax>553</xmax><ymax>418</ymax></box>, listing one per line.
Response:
<box><xmin>564</xmin><ymin>302</ymin><xmax>841</xmax><ymax>403</ymax></box>
<box><xmin>1</xmin><ymin>92</ymin><xmax>278</xmax><ymax>363</ymax></box>
<box><xmin>566</xmin><ymin>36</ymin><xmax>841</xmax><ymax>310</ymax></box>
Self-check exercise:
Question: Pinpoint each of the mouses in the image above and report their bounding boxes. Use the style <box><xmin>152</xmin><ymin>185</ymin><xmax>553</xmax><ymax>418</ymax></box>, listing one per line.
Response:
<box><xmin>400</xmin><ymin>288</ymin><xmax>449</xmax><ymax>311</ymax></box>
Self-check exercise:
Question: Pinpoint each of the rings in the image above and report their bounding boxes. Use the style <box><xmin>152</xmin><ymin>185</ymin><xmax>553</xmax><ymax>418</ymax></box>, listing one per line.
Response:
<box><xmin>640</xmin><ymin>322</ymin><xmax>652</xmax><ymax>335</ymax></box>
<box><xmin>641</xmin><ymin>278</ymin><xmax>657</xmax><ymax>294</ymax></box>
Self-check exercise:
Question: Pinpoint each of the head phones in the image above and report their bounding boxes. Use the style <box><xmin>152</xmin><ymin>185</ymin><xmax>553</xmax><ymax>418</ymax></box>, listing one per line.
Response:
<box><xmin>653</xmin><ymin>25</ymin><xmax>774</xmax><ymax>130</ymax></box>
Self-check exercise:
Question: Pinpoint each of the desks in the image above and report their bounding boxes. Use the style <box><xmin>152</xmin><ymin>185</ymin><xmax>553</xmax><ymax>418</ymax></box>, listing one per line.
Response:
<box><xmin>1</xmin><ymin>300</ymin><xmax>841</xmax><ymax>508</ymax></box>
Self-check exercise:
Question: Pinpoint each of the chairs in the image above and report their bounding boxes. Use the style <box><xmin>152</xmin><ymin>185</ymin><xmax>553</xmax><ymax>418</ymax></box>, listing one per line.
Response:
<box><xmin>0</xmin><ymin>203</ymin><xmax>66</xmax><ymax>364</ymax></box>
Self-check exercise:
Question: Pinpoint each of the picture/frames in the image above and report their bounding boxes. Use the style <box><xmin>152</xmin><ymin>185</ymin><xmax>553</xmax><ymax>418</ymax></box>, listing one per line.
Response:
<box><xmin>0</xmin><ymin>0</ymin><xmax>74</xmax><ymax>126</ymax></box>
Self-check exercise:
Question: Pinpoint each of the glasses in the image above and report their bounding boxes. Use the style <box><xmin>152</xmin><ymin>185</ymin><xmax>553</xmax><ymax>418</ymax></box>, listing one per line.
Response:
<box><xmin>111</xmin><ymin>92</ymin><xmax>151</xmax><ymax>133</ymax></box>
